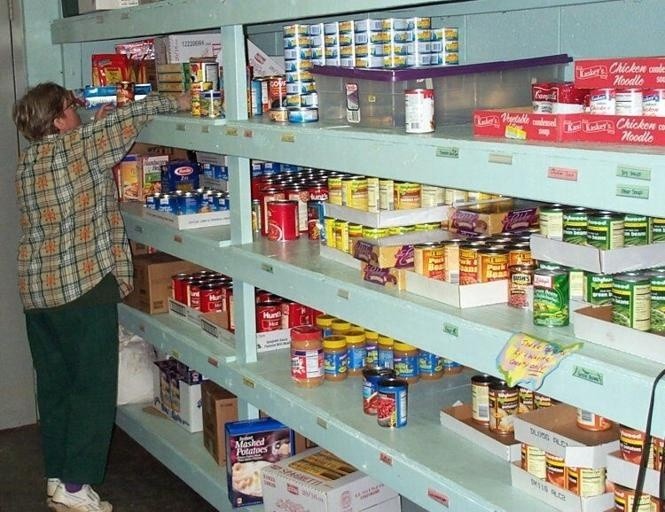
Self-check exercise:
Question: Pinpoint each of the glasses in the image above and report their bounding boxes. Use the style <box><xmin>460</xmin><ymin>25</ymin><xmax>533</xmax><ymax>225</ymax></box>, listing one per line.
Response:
<box><xmin>64</xmin><ymin>100</ymin><xmax>77</xmax><ymax>111</ymax></box>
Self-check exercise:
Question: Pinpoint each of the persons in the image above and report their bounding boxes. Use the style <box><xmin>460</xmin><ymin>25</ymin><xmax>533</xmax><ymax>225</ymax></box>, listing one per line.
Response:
<box><xmin>7</xmin><ymin>81</ymin><xmax>197</xmax><ymax>512</ymax></box>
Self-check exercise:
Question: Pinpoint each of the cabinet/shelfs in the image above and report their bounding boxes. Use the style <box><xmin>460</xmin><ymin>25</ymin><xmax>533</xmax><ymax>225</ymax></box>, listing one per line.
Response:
<box><xmin>49</xmin><ymin>0</ymin><xmax>665</xmax><ymax>512</ymax></box>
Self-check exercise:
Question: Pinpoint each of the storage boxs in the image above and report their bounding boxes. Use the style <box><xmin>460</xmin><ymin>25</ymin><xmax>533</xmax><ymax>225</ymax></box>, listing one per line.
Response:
<box><xmin>151</xmin><ymin>359</ymin><xmax>402</xmax><ymax>512</ymax></box>
<box><xmin>302</xmin><ymin>52</ymin><xmax>572</xmax><ymax>136</ymax></box>
<box><xmin>120</xmin><ymin>246</ymin><xmax>209</xmax><ymax>316</ymax></box>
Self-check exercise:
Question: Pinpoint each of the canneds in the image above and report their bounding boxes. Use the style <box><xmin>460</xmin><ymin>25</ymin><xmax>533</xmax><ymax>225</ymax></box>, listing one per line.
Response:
<box><xmin>191</xmin><ymin>81</ymin><xmax>213</xmax><ymax>117</ymax></box>
<box><xmin>540</xmin><ymin>204</ymin><xmax>665</xmax><ymax>250</ymax></box>
<box><xmin>246</xmin><ymin>17</ymin><xmax>459</xmax><ymax>125</ymax></box>
<box><xmin>377</xmin><ymin>379</ymin><xmax>407</xmax><ymax>429</ymax></box>
<box><xmin>200</xmin><ymin>89</ymin><xmax>223</xmax><ymax>119</ymax></box>
<box><xmin>323</xmin><ymin>176</ymin><xmax>514</xmax><ymax>255</ymax></box>
<box><xmin>145</xmin><ymin>186</ymin><xmax>230</xmax><ymax>215</ymax></box>
<box><xmin>531</xmin><ymin>82</ymin><xmax>665</xmax><ymax>116</ymax></box>
<box><xmin>170</xmin><ymin>266</ymin><xmax>315</xmax><ymax>333</ymax></box>
<box><xmin>290</xmin><ymin>316</ymin><xmax>464</xmax><ymax>387</ymax></box>
<box><xmin>117</xmin><ymin>81</ymin><xmax>135</xmax><ymax>109</ymax></box>
<box><xmin>470</xmin><ymin>371</ymin><xmax>665</xmax><ymax>511</ymax></box>
<box><xmin>533</xmin><ymin>261</ymin><xmax>665</xmax><ymax>333</ymax></box>
<box><xmin>413</xmin><ymin>227</ymin><xmax>539</xmax><ymax>310</ymax></box>
<box><xmin>250</xmin><ymin>159</ymin><xmax>336</xmax><ymax>242</ymax></box>
<box><xmin>362</xmin><ymin>366</ymin><xmax>396</xmax><ymax>414</ymax></box>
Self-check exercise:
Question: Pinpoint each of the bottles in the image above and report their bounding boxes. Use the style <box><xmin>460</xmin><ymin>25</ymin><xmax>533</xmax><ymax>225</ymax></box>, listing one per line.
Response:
<box><xmin>316</xmin><ymin>313</ymin><xmax>466</xmax><ymax>383</ymax></box>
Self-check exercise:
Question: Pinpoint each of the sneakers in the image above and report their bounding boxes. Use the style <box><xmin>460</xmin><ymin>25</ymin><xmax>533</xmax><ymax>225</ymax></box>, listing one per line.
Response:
<box><xmin>48</xmin><ymin>483</ymin><xmax>113</xmax><ymax>512</ymax></box>
<box><xmin>46</xmin><ymin>478</ymin><xmax>60</xmax><ymax>503</ymax></box>
